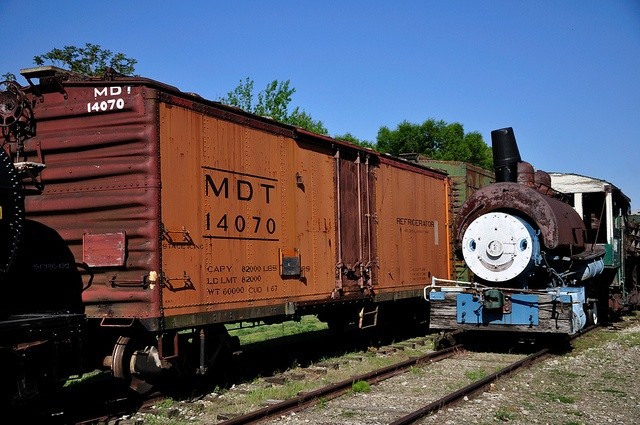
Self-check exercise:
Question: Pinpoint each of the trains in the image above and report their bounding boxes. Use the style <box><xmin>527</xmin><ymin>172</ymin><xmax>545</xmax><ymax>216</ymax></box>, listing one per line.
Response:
<box><xmin>0</xmin><ymin>65</ymin><xmax>494</xmax><ymax>425</ymax></box>
<box><xmin>425</xmin><ymin>126</ymin><xmax>633</xmax><ymax>349</ymax></box>
